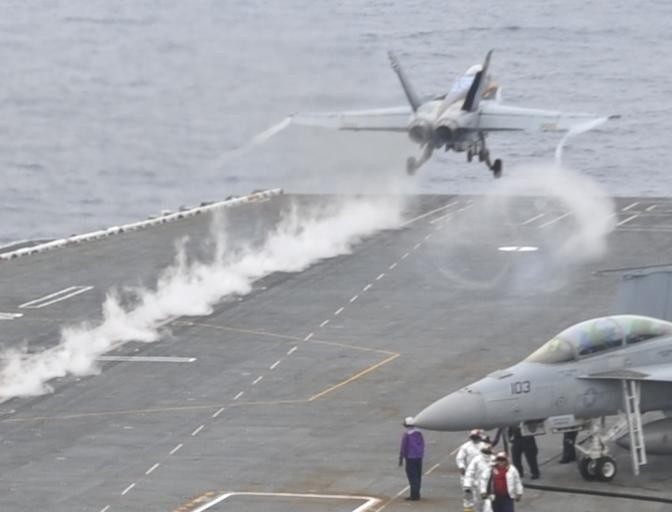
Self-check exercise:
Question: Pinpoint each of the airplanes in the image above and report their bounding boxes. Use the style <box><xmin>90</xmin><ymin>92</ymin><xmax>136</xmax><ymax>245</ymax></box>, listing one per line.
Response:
<box><xmin>286</xmin><ymin>49</ymin><xmax>609</xmax><ymax>179</ymax></box>
<box><xmin>399</xmin><ymin>315</ymin><xmax>672</xmax><ymax>482</ymax></box>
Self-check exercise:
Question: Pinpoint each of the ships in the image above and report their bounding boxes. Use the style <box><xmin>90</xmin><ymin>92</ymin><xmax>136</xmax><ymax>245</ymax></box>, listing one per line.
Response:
<box><xmin>0</xmin><ymin>180</ymin><xmax>672</xmax><ymax>512</ymax></box>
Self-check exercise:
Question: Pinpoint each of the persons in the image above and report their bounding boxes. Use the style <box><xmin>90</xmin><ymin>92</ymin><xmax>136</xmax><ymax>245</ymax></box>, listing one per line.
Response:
<box><xmin>557</xmin><ymin>431</ymin><xmax>576</xmax><ymax>464</ymax></box>
<box><xmin>397</xmin><ymin>420</ymin><xmax>425</xmax><ymax>501</ymax></box>
<box><xmin>509</xmin><ymin>425</ymin><xmax>540</xmax><ymax>480</ymax></box>
<box><xmin>479</xmin><ymin>449</ymin><xmax>524</xmax><ymax>512</ymax></box>
<box><xmin>462</xmin><ymin>444</ymin><xmax>496</xmax><ymax>511</ymax></box>
<box><xmin>456</xmin><ymin>427</ymin><xmax>490</xmax><ymax>511</ymax></box>
